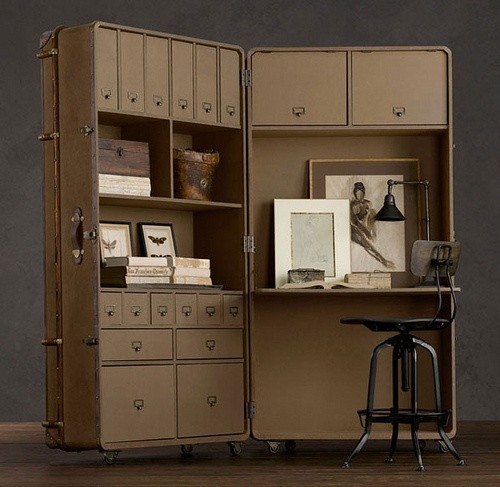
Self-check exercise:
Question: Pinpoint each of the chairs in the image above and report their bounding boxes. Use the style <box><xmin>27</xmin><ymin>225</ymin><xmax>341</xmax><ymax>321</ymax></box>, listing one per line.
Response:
<box><xmin>339</xmin><ymin>238</ymin><xmax>467</xmax><ymax>471</ymax></box>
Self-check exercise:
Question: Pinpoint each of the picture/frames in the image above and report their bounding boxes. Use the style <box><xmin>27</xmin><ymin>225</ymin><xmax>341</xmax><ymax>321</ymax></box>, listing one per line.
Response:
<box><xmin>98</xmin><ymin>219</ymin><xmax>134</xmax><ymax>266</ymax></box>
<box><xmin>306</xmin><ymin>159</ymin><xmax>422</xmax><ymax>287</ymax></box>
<box><xmin>273</xmin><ymin>198</ymin><xmax>351</xmax><ymax>288</ymax></box>
<box><xmin>137</xmin><ymin>221</ymin><xmax>179</xmax><ymax>259</ymax></box>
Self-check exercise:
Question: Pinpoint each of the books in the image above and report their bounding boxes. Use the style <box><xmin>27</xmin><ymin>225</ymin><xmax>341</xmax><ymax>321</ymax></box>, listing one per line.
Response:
<box><xmin>278</xmin><ymin>279</ymin><xmax>376</xmax><ymax>289</ymax></box>
<box><xmin>101</xmin><ymin>256</ymin><xmax>224</xmax><ymax>290</ymax></box>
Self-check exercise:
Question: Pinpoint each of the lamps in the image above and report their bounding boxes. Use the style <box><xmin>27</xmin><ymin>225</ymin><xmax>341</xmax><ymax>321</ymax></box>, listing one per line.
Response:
<box><xmin>372</xmin><ymin>179</ymin><xmax>430</xmax><ymax>241</ymax></box>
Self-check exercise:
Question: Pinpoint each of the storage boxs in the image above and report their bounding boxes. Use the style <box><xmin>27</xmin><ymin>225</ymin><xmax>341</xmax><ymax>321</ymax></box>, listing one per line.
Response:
<box><xmin>96</xmin><ymin>137</ymin><xmax>152</xmax><ymax>179</ymax></box>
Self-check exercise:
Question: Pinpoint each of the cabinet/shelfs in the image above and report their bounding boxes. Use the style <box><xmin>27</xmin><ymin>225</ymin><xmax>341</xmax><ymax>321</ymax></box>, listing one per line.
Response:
<box><xmin>40</xmin><ymin>22</ymin><xmax>248</xmax><ymax>453</ymax></box>
<box><xmin>246</xmin><ymin>45</ymin><xmax>457</xmax><ymax>445</ymax></box>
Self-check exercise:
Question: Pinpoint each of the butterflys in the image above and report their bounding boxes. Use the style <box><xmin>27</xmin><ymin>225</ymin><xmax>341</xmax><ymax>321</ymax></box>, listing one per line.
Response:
<box><xmin>147</xmin><ymin>235</ymin><xmax>167</xmax><ymax>246</ymax></box>
